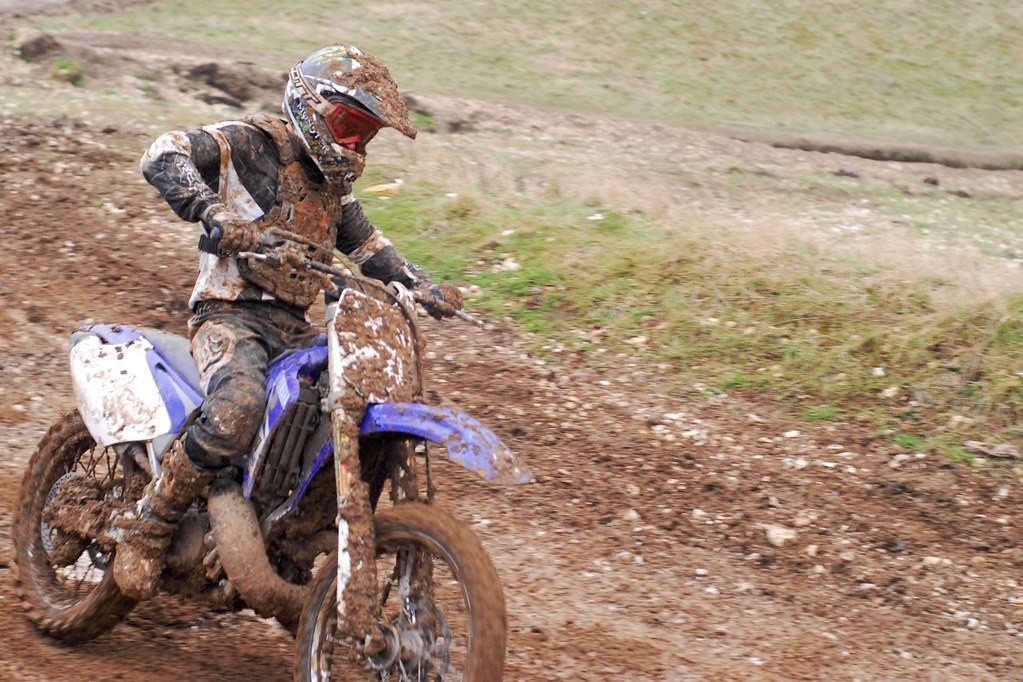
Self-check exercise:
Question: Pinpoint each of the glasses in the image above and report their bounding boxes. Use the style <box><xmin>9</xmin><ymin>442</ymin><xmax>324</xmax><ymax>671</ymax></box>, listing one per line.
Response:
<box><xmin>320</xmin><ymin>95</ymin><xmax>387</xmax><ymax>158</ymax></box>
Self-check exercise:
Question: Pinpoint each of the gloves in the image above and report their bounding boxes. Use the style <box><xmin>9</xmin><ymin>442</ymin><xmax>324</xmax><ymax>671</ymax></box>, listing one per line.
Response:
<box><xmin>203</xmin><ymin>203</ymin><xmax>264</xmax><ymax>257</ymax></box>
<box><xmin>414</xmin><ymin>281</ymin><xmax>464</xmax><ymax>320</ymax></box>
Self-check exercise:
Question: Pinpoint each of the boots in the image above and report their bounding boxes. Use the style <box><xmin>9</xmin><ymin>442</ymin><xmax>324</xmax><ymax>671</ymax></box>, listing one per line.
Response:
<box><xmin>112</xmin><ymin>434</ymin><xmax>217</xmax><ymax>602</ymax></box>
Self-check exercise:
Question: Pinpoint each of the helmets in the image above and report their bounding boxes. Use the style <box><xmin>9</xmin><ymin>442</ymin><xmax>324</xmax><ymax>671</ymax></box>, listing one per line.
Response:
<box><xmin>281</xmin><ymin>44</ymin><xmax>417</xmax><ymax>196</ymax></box>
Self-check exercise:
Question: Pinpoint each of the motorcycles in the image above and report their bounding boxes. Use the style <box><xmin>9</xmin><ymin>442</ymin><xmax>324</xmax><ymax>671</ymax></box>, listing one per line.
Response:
<box><xmin>10</xmin><ymin>228</ymin><xmax>535</xmax><ymax>680</ymax></box>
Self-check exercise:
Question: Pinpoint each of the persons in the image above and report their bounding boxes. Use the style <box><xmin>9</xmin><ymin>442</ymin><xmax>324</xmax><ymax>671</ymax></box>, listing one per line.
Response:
<box><xmin>113</xmin><ymin>44</ymin><xmax>467</xmax><ymax>603</ymax></box>
